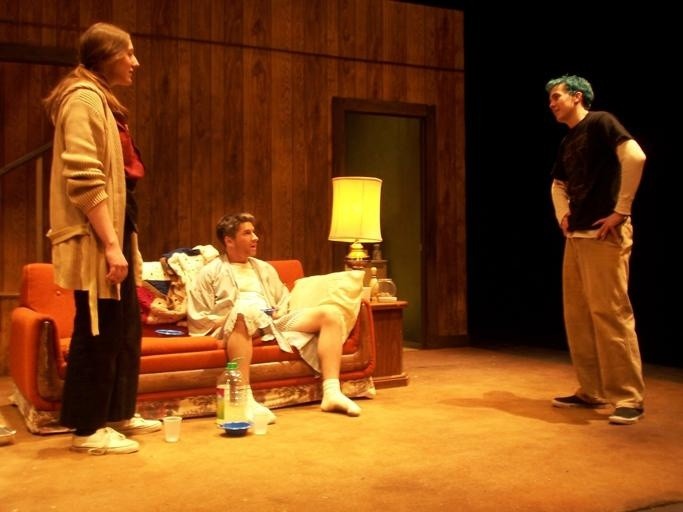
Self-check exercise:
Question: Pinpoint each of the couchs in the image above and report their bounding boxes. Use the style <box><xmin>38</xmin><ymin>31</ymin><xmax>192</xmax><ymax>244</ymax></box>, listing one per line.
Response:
<box><xmin>9</xmin><ymin>257</ymin><xmax>379</xmax><ymax>437</ymax></box>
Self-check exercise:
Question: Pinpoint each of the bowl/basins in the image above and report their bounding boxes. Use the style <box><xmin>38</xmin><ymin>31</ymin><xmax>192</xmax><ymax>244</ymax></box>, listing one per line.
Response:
<box><xmin>219</xmin><ymin>422</ymin><xmax>251</xmax><ymax>435</ymax></box>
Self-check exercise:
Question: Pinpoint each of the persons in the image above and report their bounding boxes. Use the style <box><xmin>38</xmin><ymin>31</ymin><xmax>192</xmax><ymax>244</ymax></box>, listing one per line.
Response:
<box><xmin>544</xmin><ymin>73</ymin><xmax>647</xmax><ymax>426</ymax></box>
<box><xmin>39</xmin><ymin>22</ymin><xmax>164</xmax><ymax>454</ymax></box>
<box><xmin>186</xmin><ymin>212</ymin><xmax>360</xmax><ymax>425</ymax></box>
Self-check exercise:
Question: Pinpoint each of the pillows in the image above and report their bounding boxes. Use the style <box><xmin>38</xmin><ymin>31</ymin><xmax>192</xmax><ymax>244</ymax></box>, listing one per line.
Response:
<box><xmin>287</xmin><ymin>268</ymin><xmax>367</xmax><ymax>345</ymax></box>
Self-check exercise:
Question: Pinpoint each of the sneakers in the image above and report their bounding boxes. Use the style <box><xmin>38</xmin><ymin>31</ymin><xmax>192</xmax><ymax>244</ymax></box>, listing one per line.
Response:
<box><xmin>104</xmin><ymin>416</ymin><xmax>162</xmax><ymax>435</ymax></box>
<box><xmin>608</xmin><ymin>408</ymin><xmax>646</xmax><ymax>426</ymax></box>
<box><xmin>69</xmin><ymin>425</ymin><xmax>139</xmax><ymax>455</ymax></box>
<box><xmin>551</xmin><ymin>393</ymin><xmax>612</xmax><ymax>410</ymax></box>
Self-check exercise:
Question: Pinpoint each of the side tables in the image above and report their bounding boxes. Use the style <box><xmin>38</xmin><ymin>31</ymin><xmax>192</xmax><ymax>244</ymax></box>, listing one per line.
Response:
<box><xmin>368</xmin><ymin>299</ymin><xmax>410</xmax><ymax>390</ymax></box>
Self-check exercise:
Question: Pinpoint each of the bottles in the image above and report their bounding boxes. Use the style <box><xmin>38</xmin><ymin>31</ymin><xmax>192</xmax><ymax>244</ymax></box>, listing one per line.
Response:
<box><xmin>216</xmin><ymin>362</ymin><xmax>246</xmax><ymax>428</ymax></box>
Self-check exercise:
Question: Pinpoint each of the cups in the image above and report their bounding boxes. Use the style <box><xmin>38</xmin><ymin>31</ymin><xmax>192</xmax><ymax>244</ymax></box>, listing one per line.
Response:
<box><xmin>253</xmin><ymin>410</ymin><xmax>269</xmax><ymax>435</ymax></box>
<box><xmin>162</xmin><ymin>416</ymin><xmax>183</xmax><ymax>443</ymax></box>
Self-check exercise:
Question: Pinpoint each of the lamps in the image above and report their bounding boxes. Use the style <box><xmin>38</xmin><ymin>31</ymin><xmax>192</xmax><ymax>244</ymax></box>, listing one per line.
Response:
<box><xmin>326</xmin><ymin>175</ymin><xmax>388</xmax><ymax>287</ymax></box>
